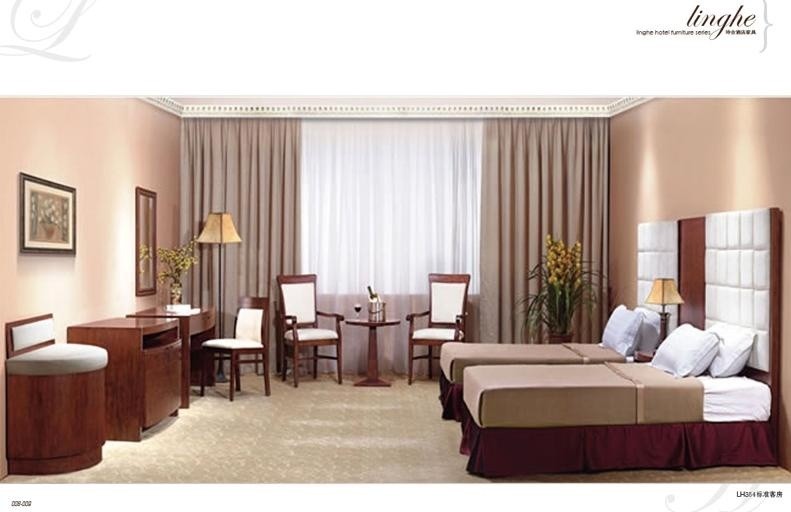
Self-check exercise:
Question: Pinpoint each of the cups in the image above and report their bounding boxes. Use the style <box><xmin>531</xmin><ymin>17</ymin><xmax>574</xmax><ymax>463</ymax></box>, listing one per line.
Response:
<box><xmin>353</xmin><ymin>303</ymin><xmax>362</xmax><ymax>320</ymax></box>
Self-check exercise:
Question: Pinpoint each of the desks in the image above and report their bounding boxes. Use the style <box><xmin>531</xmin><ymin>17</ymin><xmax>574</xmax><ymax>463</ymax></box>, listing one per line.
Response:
<box><xmin>126</xmin><ymin>305</ymin><xmax>217</xmax><ymax>407</ymax></box>
<box><xmin>345</xmin><ymin>319</ymin><xmax>401</xmax><ymax>387</ymax></box>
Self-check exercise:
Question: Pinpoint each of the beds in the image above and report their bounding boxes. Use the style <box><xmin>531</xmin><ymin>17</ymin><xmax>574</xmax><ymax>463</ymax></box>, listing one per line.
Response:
<box><xmin>441</xmin><ymin>221</ymin><xmax>682</xmax><ymax>424</ymax></box>
<box><xmin>462</xmin><ymin>207</ymin><xmax>782</xmax><ymax>476</ymax></box>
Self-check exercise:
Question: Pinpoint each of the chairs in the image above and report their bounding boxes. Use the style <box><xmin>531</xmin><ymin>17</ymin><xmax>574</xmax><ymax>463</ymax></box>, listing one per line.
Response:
<box><xmin>200</xmin><ymin>296</ymin><xmax>270</xmax><ymax>401</ymax></box>
<box><xmin>277</xmin><ymin>275</ymin><xmax>344</xmax><ymax>388</ymax></box>
<box><xmin>405</xmin><ymin>274</ymin><xmax>471</xmax><ymax>385</ymax></box>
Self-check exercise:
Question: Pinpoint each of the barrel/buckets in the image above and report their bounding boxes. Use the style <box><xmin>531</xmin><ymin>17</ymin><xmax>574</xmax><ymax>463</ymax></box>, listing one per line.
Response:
<box><xmin>368</xmin><ymin>302</ymin><xmax>386</xmax><ymax>322</ymax></box>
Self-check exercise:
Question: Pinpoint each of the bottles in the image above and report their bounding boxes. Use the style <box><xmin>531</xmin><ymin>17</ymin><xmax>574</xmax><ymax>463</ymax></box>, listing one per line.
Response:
<box><xmin>367</xmin><ymin>286</ymin><xmax>377</xmax><ymax>301</ymax></box>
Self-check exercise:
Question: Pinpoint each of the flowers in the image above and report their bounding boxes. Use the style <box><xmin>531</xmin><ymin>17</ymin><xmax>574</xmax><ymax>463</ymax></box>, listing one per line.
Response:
<box><xmin>157</xmin><ymin>235</ymin><xmax>199</xmax><ymax>283</ymax></box>
<box><xmin>139</xmin><ymin>243</ymin><xmax>147</xmax><ymax>274</ymax></box>
<box><xmin>512</xmin><ymin>235</ymin><xmax>609</xmax><ymax>343</ymax></box>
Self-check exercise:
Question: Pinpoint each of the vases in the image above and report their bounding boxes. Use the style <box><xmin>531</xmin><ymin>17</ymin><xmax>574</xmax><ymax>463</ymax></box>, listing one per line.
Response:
<box><xmin>169</xmin><ymin>282</ymin><xmax>181</xmax><ymax>305</ymax></box>
<box><xmin>547</xmin><ymin>335</ymin><xmax>573</xmax><ymax>344</ymax></box>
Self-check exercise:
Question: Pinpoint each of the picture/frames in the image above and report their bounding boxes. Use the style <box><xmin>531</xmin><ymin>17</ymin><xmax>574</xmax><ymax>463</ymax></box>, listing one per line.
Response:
<box><xmin>18</xmin><ymin>173</ymin><xmax>77</xmax><ymax>256</ymax></box>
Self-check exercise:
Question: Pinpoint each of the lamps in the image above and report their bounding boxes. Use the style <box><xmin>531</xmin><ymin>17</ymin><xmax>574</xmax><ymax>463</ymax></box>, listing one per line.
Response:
<box><xmin>646</xmin><ymin>278</ymin><xmax>685</xmax><ymax>356</ymax></box>
<box><xmin>193</xmin><ymin>213</ymin><xmax>242</xmax><ymax>382</ymax></box>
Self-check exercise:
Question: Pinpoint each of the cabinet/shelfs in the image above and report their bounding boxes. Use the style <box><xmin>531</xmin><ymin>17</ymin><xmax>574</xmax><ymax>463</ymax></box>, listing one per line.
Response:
<box><xmin>67</xmin><ymin>317</ymin><xmax>182</xmax><ymax>442</ymax></box>
<box><xmin>6</xmin><ymin>312</ymin><xmax>109</xmax><ymax>476</ymax></box>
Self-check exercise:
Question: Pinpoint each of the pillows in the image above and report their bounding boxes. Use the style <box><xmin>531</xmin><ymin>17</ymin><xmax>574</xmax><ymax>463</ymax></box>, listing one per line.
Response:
<box><xmin>635</xmin><ymin>309</ymin><xmax>661</xmax><ymax>357</ymax></box>
<box><xmin>598</xmin><ymin>303</ymin><xmax>643</xmax><ymax>357</ymax></box>
<box><xmin>705</xmin><ymin>323</ymin><xmax>756</xmax><ymax>378</ymax></box>
<box><xmin>650</xmin><ymin>322</ymin><xmax>718</xmax><ymax>378</ymax></box>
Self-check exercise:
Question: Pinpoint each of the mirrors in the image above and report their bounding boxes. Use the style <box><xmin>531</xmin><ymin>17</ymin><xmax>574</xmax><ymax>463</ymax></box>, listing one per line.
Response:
<box><xmin>134</xmin><ymin>186</ymin><xmax>157</xmax><ymax>296</ymax></box>
